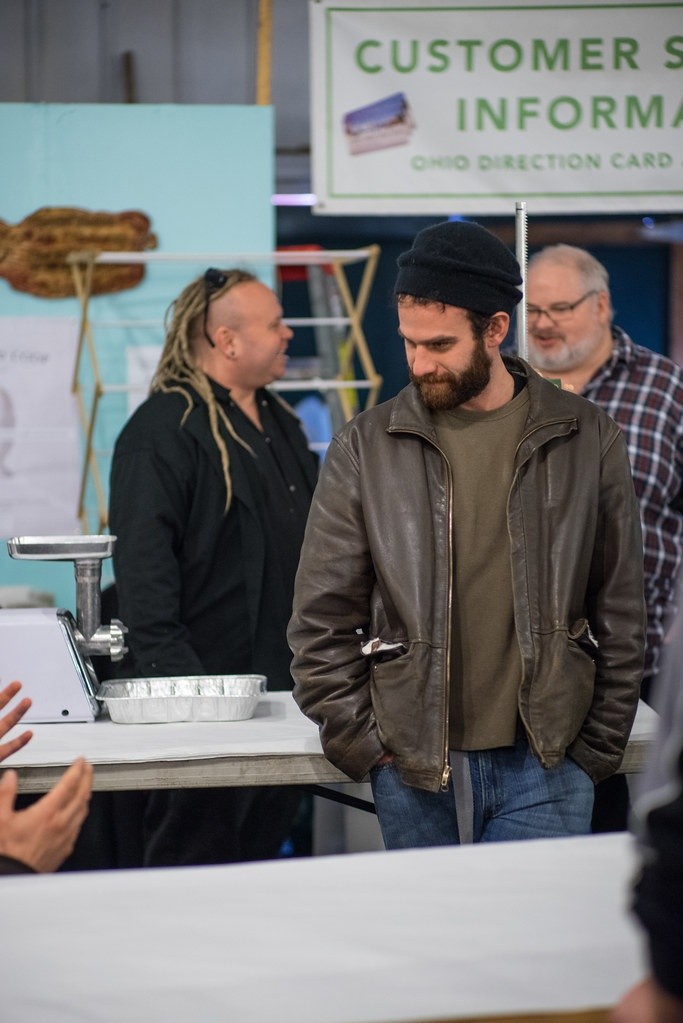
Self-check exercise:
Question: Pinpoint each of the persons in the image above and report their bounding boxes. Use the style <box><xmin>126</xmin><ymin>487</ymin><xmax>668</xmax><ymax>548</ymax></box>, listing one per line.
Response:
<box><xmin>108</xmin><ymin>268</ymin><xmax>322</xmax><ymax>869</ymax></box>
<box><xmin>616</xmin><ymin>583</ymin><xmax>682</xmax><ymax>1023</ymax></box>
<box><xmin>1</xmin><ymin>682</ymin><xmax>95</xmax><ymax>876</ymax></box>
<box><xmin>516</xmin><ymin>243</ymin><xmax>683</xmax><ymax>703</ymax></box>
<box><xmin>285</xmin><ymin>222</ymin><xmax>648</xmax><ymax>850</ymax></box>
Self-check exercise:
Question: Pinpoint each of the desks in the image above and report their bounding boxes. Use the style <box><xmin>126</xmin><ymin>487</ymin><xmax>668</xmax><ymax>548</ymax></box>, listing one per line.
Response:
<box><xmin>0</xmin><ymin>690</ymin><xmax>662</xmax><ymax>856</ymax></box>
<box><xmin>0</xmin><ymin>832</ymin><xmax>650</xmax><ymax>1023</ymax></box>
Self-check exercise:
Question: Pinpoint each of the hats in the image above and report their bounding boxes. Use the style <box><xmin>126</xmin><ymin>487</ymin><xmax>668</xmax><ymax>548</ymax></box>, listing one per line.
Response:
<box><xmin>393</xmin><ymin>218</ymin><xmax>524</xmax><ymax>318</ymax></box>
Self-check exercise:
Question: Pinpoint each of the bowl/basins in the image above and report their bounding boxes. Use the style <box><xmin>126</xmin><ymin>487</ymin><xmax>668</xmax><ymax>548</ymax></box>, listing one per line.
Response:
<box><xmin>95</xmin><ymin>674</ymin><xmax>268</xmax><ymax>724</ymax></box>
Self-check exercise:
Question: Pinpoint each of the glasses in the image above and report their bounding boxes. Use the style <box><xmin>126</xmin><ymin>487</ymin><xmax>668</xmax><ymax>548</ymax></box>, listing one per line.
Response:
<box><xmin>525</xmin><ymin>290</ymin><xmax>595</xmax><ymax>325</ymax></box>
<box><xmin>202</xmin><ymin>268</ymin><xmax>228</xmax><ymax>349</ymax></box>
